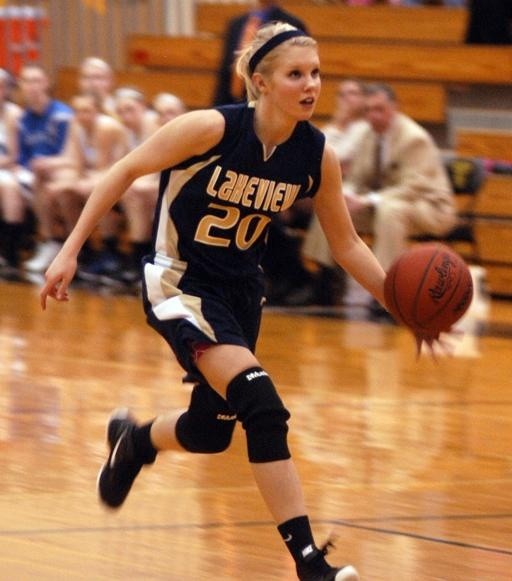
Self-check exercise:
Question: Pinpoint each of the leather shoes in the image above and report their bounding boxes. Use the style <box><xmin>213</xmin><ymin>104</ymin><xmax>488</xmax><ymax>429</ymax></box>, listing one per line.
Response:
<box><xmin>367</xmin><ymin>301</ymin><xmax>387</xmax><ymax>315</ymax></box>
<box><xmin>282</xmin><ymin>275</ymin><xmax>330</xmax><ymax>306</ymax></box>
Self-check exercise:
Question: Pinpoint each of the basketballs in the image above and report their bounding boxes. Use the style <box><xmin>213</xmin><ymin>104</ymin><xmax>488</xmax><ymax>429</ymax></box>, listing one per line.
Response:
<box><xmin>384</xmin><ymin>242</ymin><xmax>474</xmax><ymax>336</ymax></box>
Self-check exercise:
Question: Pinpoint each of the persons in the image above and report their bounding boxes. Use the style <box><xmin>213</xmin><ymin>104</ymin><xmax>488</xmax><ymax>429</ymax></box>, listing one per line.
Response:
<box><xmin>38</xmin><ymin>17</ymin><xmax>469</xmax><ymax>580</ymax></box>
<box><xmin>209</xmin><ymin>2</ymin><xmax>307</xmax><ymax>110</ymax></box>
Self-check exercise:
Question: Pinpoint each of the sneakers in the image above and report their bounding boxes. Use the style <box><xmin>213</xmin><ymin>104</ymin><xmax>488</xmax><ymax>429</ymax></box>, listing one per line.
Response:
<box><xmin>96</xmin><ymin>408</ymin><xmax>144</xmax><ymax>507</ymax></box>
<box><xmin>295</xmin><ymin>542</ymin><xmax>360</xmax><ymax>581</ymax></box>
<box><xmin>2</xmin><ymin>230</ymin><xmax>149</xmax><ymax>286</ymax></box>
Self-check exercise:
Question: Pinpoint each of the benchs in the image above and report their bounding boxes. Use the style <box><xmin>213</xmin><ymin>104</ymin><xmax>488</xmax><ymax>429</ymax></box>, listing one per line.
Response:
<box><xmin>17</xmin><ymin>2</ymin><xmax>511</xmax><ymax>339</ymax></box>
